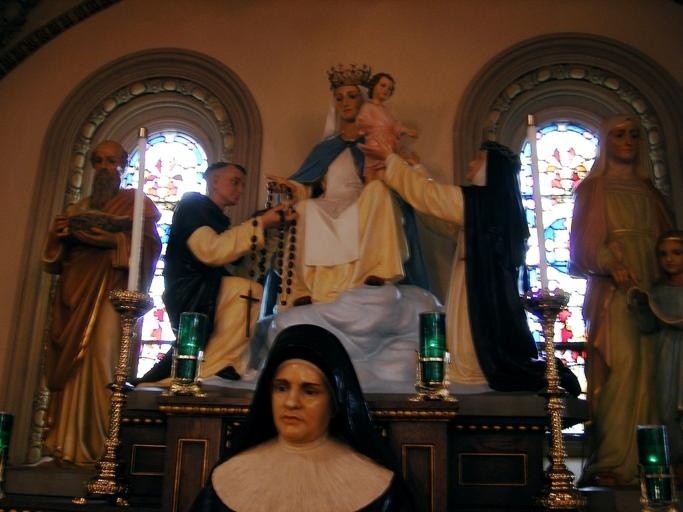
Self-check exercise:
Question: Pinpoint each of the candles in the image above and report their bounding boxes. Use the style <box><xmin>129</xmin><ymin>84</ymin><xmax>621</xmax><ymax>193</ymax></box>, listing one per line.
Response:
<box><xmin>125</xmin><ymin>125</ymin><xmax>147</xmax><ymax>293</ymax></box>
<box><xmin>525</xmin><ymin>112</ymin><xmax>551</xmax><ymax>291</ymax></box>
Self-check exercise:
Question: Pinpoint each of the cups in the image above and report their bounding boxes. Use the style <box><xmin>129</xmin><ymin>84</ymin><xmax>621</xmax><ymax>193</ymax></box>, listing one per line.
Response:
<box><xmin>416</xmin><ymin>310</ymin><xmax>446</xmax><ymax>382</ymax></box>
<box><xmin>633</xmin><ymin>424</ymin><xmax>672</xmax><ymax>511</ymax></box>
<box><xmin>0</xmin><ymin>412</ymin><xmax>15</xmax><ymax>499</ymax></box>
<box><xmin>173</xmin><ymin>312</ymin><xmax>211</xmax><ymax>381</ymax></box>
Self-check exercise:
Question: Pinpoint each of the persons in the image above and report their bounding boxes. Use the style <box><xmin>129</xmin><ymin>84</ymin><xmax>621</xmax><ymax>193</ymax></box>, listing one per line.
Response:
<box><xmin>571</xmin><ymin>111</ymin><xmax>675</xmax><ymax>490</ymax></box>
<box><xmin>37</xmin><ymin>138</ymin><xmax>161</xmax><ymax>475</ymax></box>
<box><xmin>185</xmin><ymin>324</ymin><xmax>404</xmax><ymax>512</ymax></box>
<box><xmin>355</xmin><ymin>69</ymin><xmax>419</xmax><ymax>177</ymax></box>
<box><xmin>356</xmin><ymin>135</ymin><xmax>581</xmax><ymax>401</ymax></box>
<box><xmin>267</xmin><ymin>65</ymin><xmax>427</xmax><ymax>310</ymax></box>
<box><xmin>159</xmin><ymin>161</ymin><xmax>299</xmax><ymax>379</ymax></box>
<box><xmin>623</xmin><ymin>227</ymin><xmax>682</xmax><ymax>460</ymax></box>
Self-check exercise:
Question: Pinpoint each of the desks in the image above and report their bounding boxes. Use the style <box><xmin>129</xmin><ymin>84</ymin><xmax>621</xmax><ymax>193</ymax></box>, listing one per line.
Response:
<box><xmin>97</xmin><ymin>378</ymin><xmax>577</xmax><ymax>512</ymax></box>
